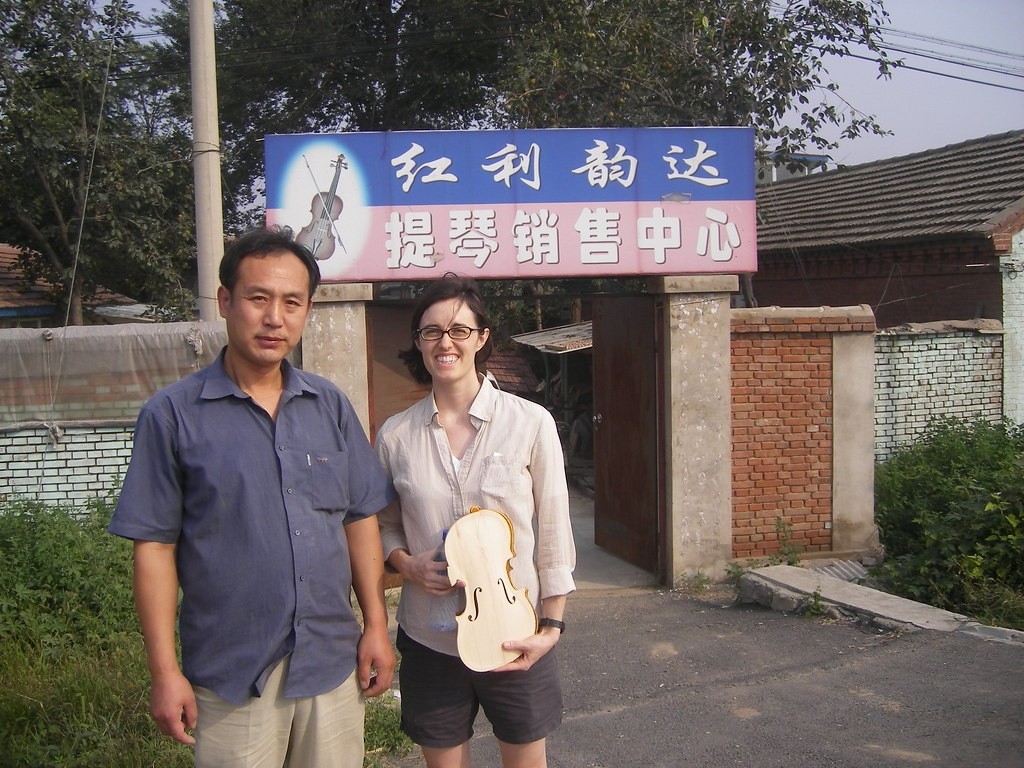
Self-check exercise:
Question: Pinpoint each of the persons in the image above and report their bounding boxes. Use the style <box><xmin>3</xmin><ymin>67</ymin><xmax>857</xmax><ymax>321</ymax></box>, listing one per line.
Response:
<box><xmin>374</xmin><ymin>282</ymin><xmax>575</xmax><ymax>768</ymax></box>
<box><xmin>108</xmin><ymin>229</ymin><xmax>397</xmax><ymax>768</ymax></box>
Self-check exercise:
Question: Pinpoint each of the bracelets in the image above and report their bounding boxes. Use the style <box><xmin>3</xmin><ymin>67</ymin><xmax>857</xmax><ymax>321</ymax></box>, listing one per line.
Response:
<box><xmin>539</xmin><ymin>618</ymin><xmax>565</xmax><ymax>633</ymax></box>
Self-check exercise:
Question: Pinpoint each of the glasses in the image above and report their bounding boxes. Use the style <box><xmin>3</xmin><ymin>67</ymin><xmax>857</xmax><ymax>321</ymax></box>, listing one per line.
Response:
<box><xmin>416</xmin><ymin>327</ymin><xmax>481</xmax><ymax>341</ymax></box>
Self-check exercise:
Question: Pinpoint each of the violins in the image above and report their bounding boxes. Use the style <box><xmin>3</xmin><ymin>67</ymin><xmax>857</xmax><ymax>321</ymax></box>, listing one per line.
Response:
<box><xmin>293</xmin><ymin>154</ymin><xmax>349</xmax><ymax>261</ymax></box>
